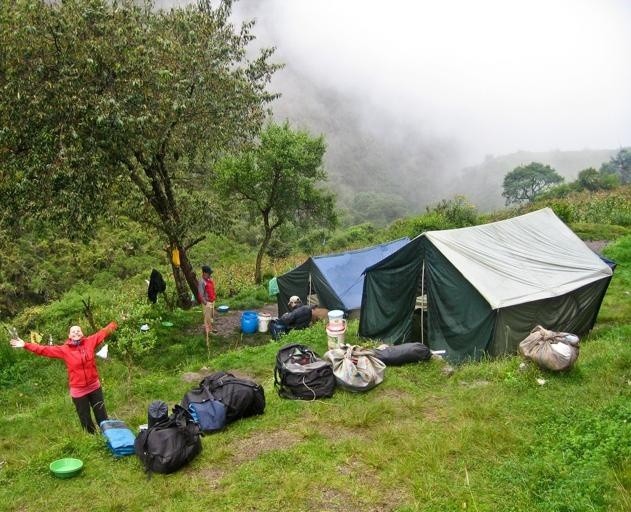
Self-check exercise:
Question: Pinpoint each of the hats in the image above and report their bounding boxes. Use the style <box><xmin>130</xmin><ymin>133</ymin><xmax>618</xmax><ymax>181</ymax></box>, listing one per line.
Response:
<box><xmin>202</xmin><ymin>265</ymin><xmax>213</xmax><ymax>273</ymax></box>
<box><xmin>287</xmin><ymin>295</ymin><xmax>300</xmax><ymax>305</ymax></box>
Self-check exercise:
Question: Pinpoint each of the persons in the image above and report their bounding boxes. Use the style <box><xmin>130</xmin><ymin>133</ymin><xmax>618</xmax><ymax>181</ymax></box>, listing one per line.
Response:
<box><xmin>198</xmin><ymin>265</ymin><xmax>220</xmax><ymax>338</ymax></box>
<box><xmin>268</xmin><ymin>295</ymin><xmax>313</xmax><ymax>342</ymax></box>
<box><xmin>9</xmin><ymin>313</ymin><xmax>129</xmax><ymax>434</ymax></box>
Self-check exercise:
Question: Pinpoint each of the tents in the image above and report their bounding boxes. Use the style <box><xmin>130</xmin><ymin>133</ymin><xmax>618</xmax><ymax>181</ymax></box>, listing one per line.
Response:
<box><xmin>275</xmin><ymin>234</ymin><xmax>413</xmax><ymax>325</ymax></box>
<box><xmin>360</xmin><ymin>206</ymin><xmax>615</xmax><ymax>368</ymax></box>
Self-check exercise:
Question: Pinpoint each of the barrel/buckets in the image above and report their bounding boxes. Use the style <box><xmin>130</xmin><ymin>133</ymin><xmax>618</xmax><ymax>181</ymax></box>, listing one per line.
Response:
<box><xmin>240</xmin><ymin>312</ymin><xmax>258</xmax><ymax>334</ymax></box>
<box><xmin>258</xmin><ymin>313</ymin><xmax>272</xmax><ymax>333</ymax></box>
<box><xmin>328</xmin><ymin>310</ymin><xmax>345</xmax><ymax>332</ymax></box>
<box><xmin>325</xmin><ymin>330</ymin><xmax>346</xmax><ymax>351</ymax></box>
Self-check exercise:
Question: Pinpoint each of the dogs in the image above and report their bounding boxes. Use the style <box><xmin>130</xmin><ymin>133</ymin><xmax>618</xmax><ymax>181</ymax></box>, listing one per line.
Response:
<box><xmin>287</xmin><ymin>296</ymin><xmax>330</xmax><ymax>323</ymax></box>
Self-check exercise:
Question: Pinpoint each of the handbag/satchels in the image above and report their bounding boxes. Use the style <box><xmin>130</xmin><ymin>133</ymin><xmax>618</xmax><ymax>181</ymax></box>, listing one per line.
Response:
<box><xmin>134</xmin><ymin>371</ymin><xmax>265</xmax><ymax>473</ymax></box>
<box><xmin>274</xmin><ymin>342</ymin><xmax>431</xmax><ymax>399</ymax></box>
